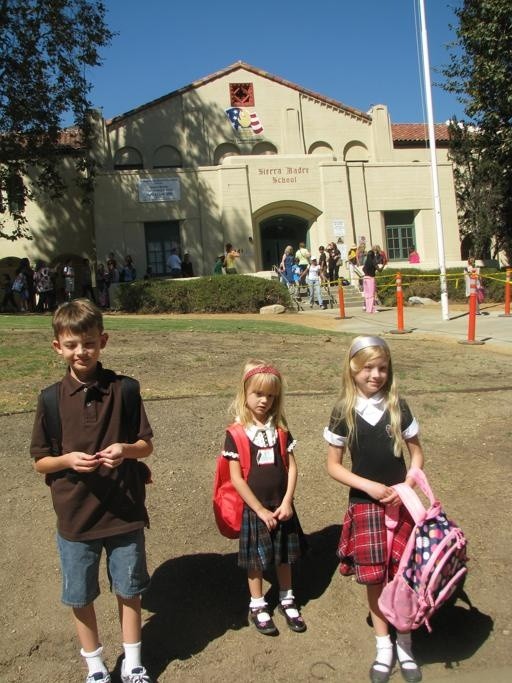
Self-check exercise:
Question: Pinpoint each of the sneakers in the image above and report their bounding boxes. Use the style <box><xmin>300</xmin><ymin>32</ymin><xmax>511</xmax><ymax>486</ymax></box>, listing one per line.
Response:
<box><xmin>120</xmin><ymin>658</ymin><xmax>151</xmax><ymax>682</ymax></box>
<box><xmin>86</xmin><ymin>669</ymin><xmax>113</xmax><ymax>683</ymax></box>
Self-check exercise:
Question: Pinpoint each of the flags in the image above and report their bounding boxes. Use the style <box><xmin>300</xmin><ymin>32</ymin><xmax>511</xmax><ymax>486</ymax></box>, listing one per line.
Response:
<box><xmin>224</xmin><ymin>107</ymin><xmax>264</xmax><ymax>135</ymax></box>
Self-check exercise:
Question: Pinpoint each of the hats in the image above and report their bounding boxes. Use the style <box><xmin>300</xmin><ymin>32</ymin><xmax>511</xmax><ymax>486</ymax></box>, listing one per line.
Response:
<box><xmin>311</xmin><ymin>256</ymin><xmax>317</xmax><ymax>261</ymax></box>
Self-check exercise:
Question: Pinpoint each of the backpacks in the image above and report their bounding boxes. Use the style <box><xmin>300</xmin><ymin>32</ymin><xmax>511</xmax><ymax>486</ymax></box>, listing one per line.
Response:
<box><xmin>377</xmin><ymin>463</ymin><xmax>468</xmax><ymax>633</ymax></box>
<box><xmin>211</xmin><ymin>420</ymin><xmax>290</xmax><ymax>539</ymax></box>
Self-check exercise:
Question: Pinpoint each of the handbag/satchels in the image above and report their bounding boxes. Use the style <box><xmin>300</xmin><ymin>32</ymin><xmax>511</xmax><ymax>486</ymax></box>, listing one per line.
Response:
<box><xmin>335</xmin><ymin>257</ymin><xmax>343</xmax><ymax>267</ymax></box>
<box><xmin>11</xmin><ymin>272</ymin><xmax>27</xmax><ymax>293</ymax></box>
<box><xmin>476</xmin><ymin>286</ymin><xmax>486</xmax><ymax>304</ymax></box>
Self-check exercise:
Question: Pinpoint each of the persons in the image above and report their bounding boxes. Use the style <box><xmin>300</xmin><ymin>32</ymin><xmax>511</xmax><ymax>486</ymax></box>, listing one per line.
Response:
<box><xmin>348</xmin><ymin>236</ymin><xmax>387</xmax><ymax>312</ymax></box>
<box><xmin>408</xmin><ymin>244</ymin><xmax>420</xmax><ymax>263</ymax></box>
<box><xmin>29</xmin><ymin>297</ymin><xmax>162</xmax><ymax>683</ymax></box>
<box><xmin>463</xmin><ymin>257</ymin><xmax>484</xmax><ymax>316</ymax></box>
<box><xmin>144</xmin><ymin>249</ymin><xmax>194</xmax><ymax>280</ymax></box>
<box><xmin>0</xmin><ymin>252</ymin><xmax>136</xmax><ymax>313</ymax></box>
<box><xmin>221</xmin><ymin>356</ymin><xmax>307</xmax><ymax>636</ymax></box>
<box><xmin>278</xmin><ymin>237</ymin><xmax>346</xmax><ymax>310</ymax></box>
<box><xmin>214</xmin><ymin>244</ymin><xmax>240</xmax><ymax>274</ymax></box>
<box><xmin>323</xmin><ymin>334</ymin><xmax>426</xmax><ymax>683</ymax></box>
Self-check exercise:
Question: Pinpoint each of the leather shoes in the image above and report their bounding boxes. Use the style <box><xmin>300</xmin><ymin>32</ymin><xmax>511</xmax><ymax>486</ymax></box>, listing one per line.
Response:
<box><xmin>247</xmin><ymin>604</ymin><xmax>280</xmax><ymax>636</ymax></box>
<box><xmin>393</xmin><ymin>640</ymin><xmax>422</xmax><ymax>683</ymax></box>
<box><xmin>277</xmin><ymin>598</ymin><xmax>307</xmax><ymax>632</ymax></box>
<box><xmin>367</xmin><ymin>638</ymin><xmax>396</xmax><ymax>682</ymax></box>
